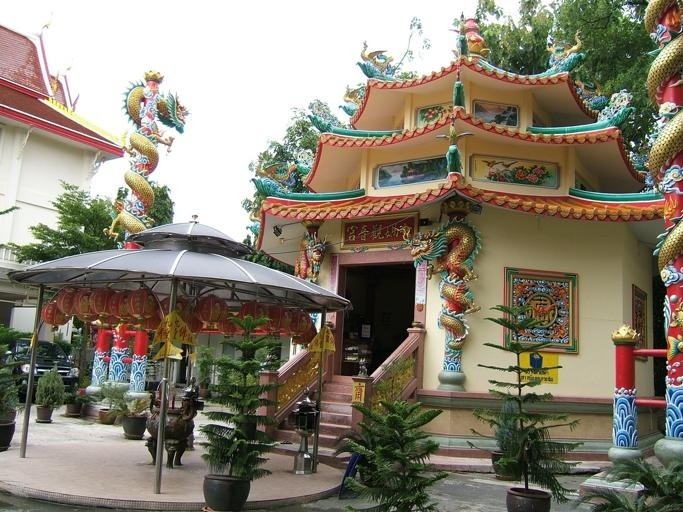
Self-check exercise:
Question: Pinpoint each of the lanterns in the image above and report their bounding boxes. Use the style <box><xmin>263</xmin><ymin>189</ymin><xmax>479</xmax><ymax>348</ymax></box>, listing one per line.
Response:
<box><xmin>41</xmin><ymin>285</ymin><xmax>319</xmax><ymax>349</ymax></box>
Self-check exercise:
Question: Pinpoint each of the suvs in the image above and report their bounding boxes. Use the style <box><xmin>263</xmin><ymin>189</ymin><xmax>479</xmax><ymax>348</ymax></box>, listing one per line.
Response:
<box><xmin>7</xmin><ymin>338</ymin><xmax>81</xmax><ymax>402</ymax></box>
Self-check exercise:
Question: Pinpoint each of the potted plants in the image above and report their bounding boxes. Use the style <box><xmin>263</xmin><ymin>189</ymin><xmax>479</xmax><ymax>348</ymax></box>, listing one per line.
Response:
<box><xmin>195</xmin><ymin>314</ymin><xmax>287</xmax><ymax>512</ymax></box>
<box><xmin>0</xmin><ymin>322</ymin><xmax>151</xmax><ymax>452</ymax></box>
<box><xmin>466</xmin><ymin>304</ymin><xmax>584</xmax><ymax>512</ymax></box>
